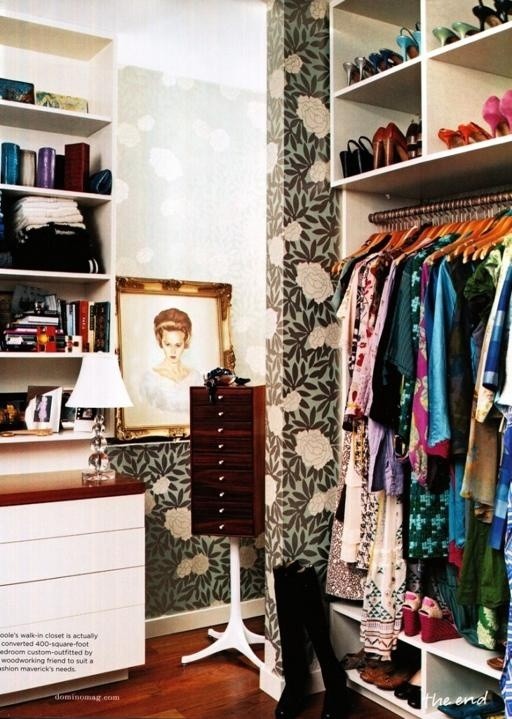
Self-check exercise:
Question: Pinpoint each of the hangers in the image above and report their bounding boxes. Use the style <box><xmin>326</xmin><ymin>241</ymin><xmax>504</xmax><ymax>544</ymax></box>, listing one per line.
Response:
<box><xmin>331</xmin><ymin>191</ymin><xmax>512</xmax><ymax>273</ymax></box>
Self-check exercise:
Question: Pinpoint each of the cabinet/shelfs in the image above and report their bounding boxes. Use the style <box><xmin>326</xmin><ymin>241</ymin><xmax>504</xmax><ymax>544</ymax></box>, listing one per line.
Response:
<box><xmin>328</xmin><ymin>0</ymin><xmax>510</xmax><ymax>713</ymax></box>
<box><xmin>0</xmin><ymin>9</ymin><xmax>154</xmax><ymax>711</ymax></box>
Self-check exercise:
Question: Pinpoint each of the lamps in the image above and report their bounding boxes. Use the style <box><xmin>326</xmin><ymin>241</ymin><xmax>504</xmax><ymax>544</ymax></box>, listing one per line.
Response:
<box><xmin>63</xmin><ymin>351</ymin><xmax>138</xmax><ymax>482</ymax></box>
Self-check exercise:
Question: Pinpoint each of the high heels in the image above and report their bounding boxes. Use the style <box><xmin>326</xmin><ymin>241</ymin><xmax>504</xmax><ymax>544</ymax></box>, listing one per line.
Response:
<box><xmin>499</xmin><ymin>90</ymin><xmax>512</xmax><ymax>135</ymax></box>
<box><xmin>372</xmin><ymin>127</ymin><xmax>386</xmax><ymax>169</ymax></box>
<box><xmin>342</xmin><ymin>60</ymin><xmax>365</xmax><ymax>87</ymax></box>
<box><xmin>339</xmin><ymin>140</ymin><xmax>363</xmax><ymax>178</ymax></box>
<box><xmin>354</xmin><ymin>56</ymin><xmax>379</xmax><ymax>82</ymax></box>
<box><xmin>481</xmin><ymin>96</ymin><xmax>510</xmax><ymax>138</ymax></box>
<box><xmin>452</xmin><ymin>21</ymin><xmax>480</xmax><ymax>40</ymax></box>
<box><xmin>382</xmin><ymin>122</ymin><xmax>409</xmax><ymax>166</ymax></box>
<box><xmin>402</xmin><ymin>591</ymin><xmax>422</xmax><ymax>637</ymax></box>
<box><xmin>405</xmin><ymin>123</ymin><xmax>418</xmax><ymax>160</ymax></box>
<box><xmin>432</xmin><ymin>25</ymin><xmax>460</xmax><ymax>48</ymax></box>
<box><xmin>352</xmin><ymin>136</ymin><xmax>373</xmax><ymax>173</ymax></box>
<box><xmin>458</xmin><ymin>121</ymin><xmax>493</xmax><ymax>146</ymax></box>
<box><xmin>395</xmin><ymin>26</ymin><xmax>418</xmax><ymax>63</ymax></box>
<box><xmin>417</xmin><ymin>596</ymin><xmax>462</xmax><ymax>643</ymax></box>
<box><xmin>379</xmin><ymin>47</ymin><xmax>403</xmax><ymax>70</ymax></box>
<box><xmin>437</xmin><ymin>128</ymin><xmax>462</xmax><ymax>150</ymax></box>
<box><xmin>368</xmin><ymin>52</ymin><xmax>393</xmax><ymax>75</ymax></box>
<box><xmin>471</xmin><ymin>0</ymin><xmax>505</xmax><ymax>32</ymax></box>
<box><xmin>493</xmin><ymin>0</ymin><xmax>512</xmax><ymax>23</ymax></box>
<box><xmin>412</xmin><ymin>22</ymin><xmax>420</xmax><ymax>53</ymax></box>
<box><xmin>415</xmin><ymin>120</ymin><xmax>422</xmax><ymax>158</ymax></box>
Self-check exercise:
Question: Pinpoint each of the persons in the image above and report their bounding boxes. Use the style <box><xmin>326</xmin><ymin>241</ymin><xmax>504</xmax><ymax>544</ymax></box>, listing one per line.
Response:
<box><xmin>126</xmin><ymin>306</ymin><xmax>209</xmax><ymax>426</ymax></box>
<box><xmin>2</xmin><ymin>398</ymin><xmax>33</xmax><ymax>428</ymax></box>
<box><xmin>35</xmin><ymin>395</ymin><xmax>50</xmax><ymax>425</ymax></box>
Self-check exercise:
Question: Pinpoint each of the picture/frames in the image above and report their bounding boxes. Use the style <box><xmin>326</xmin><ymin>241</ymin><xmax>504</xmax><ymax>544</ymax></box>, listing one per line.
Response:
<box><xmin>74</xmin><ymin>406</ymin><xmax>103</xmax><ymax>432</ymax></box>
<box><xmin>61</xmin><ymin>389</ymin><xmax>75</xmax><ymax>429</ymax></box>
<box><xmin>0</xmin><ymin>392</ymin><xmax>29</xmax><ymax>431</ymax></box>
<box><xmin>23</xmin><ymin>386</ymin><xmax>62</xmax><ymax>433</ymax></box>
<box><xmin>116</xmin><ymin>274</ymin><xmax>239</xmax><ymax>446</ymax></box>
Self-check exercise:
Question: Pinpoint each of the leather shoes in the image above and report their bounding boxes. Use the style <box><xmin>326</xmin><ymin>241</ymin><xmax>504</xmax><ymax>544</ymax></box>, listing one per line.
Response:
<box><xmin>374</xmin><ymin>671</ymin><xmax>414</xmax><ymax>692</ymax></box>
<box><xmin>393</xmin><ymin>668</ymin><xmax>420</xmax><ymax>700</ymax></box>
<box><xmin>360</xmin><ymin>664</ymin><xmax>394</xmax><ymax>684</ymax></box>
<box><xmin>407</xmin><ymin>690</ymin><xmax>421</xmax><ymax>710</ymax></box>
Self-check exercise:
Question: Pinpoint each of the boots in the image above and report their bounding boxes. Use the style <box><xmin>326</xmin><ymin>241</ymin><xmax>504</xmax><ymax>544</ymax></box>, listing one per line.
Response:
<box><xmin>297</xmin><ymin>566</ymin><xmax>356</xmax><ymax>719</ymax></box>
<box><xmin>271</xmin><ymin>564</ymin><xmax>312</xmax><ymax>719</ymax></box>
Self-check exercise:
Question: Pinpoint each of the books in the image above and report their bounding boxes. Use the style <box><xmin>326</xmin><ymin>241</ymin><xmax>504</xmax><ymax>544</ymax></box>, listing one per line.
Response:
<box><xmin>0</xmin><ymin>299</ymin><xmax>71</xmax><ymax>351</ymax></box>
<box><xmin>71</xmin><ymin>299</ymin><xmax>88</xmax><ymax>352</ymax></box>
<box><xmin>88</xmin><ymin>300</ymin><xmax>95</xmax><ymax>351</ymax></box>
<box><xmin>96</xmin><ymin>301</ymin><xmax>112</xmax><ymax>351</ymax></box>
